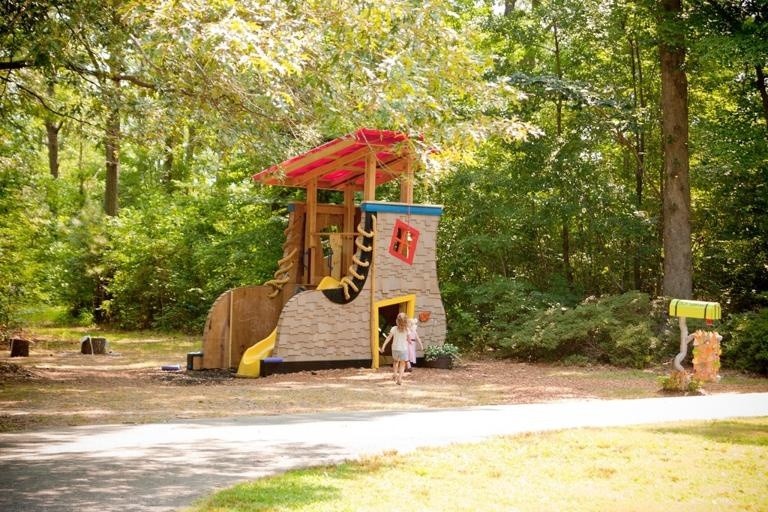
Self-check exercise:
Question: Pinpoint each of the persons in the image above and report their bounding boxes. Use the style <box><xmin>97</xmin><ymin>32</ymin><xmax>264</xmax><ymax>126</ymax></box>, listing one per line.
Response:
<box><xmin>404</xmin><ymin>318</ymin><xmax>423</xmax><ymax>371</ymax></box>
<box><xmin>380</xmin><ymin>312</ymin><xmax>412</xmax><ymax>385</ymax></box>
